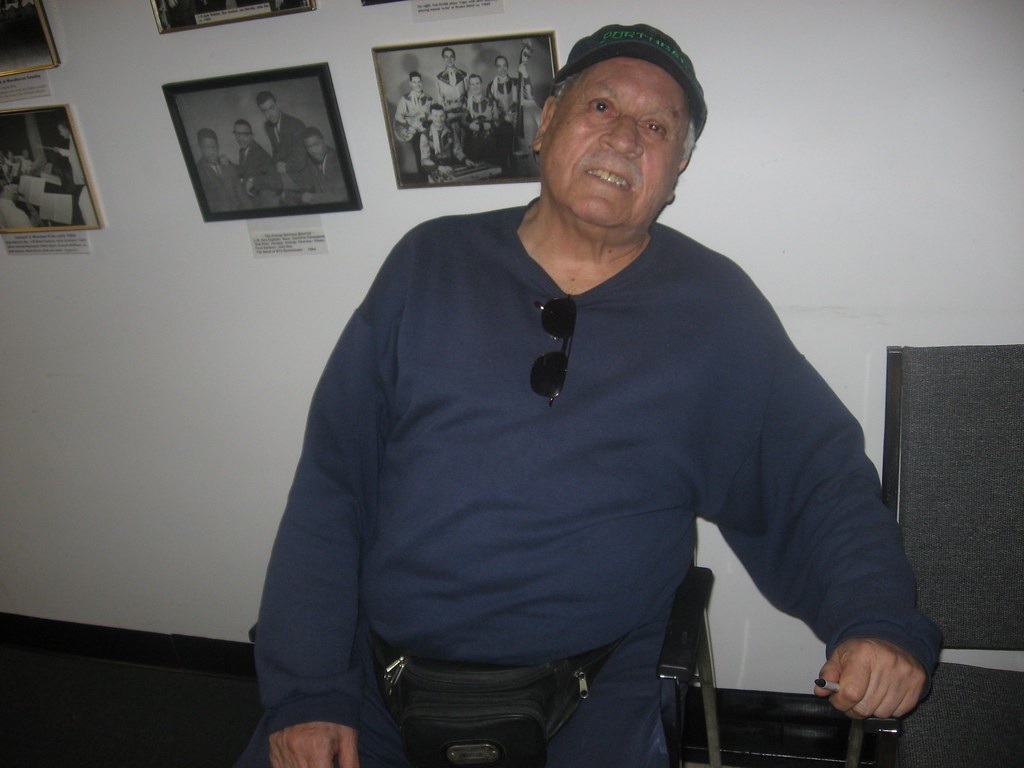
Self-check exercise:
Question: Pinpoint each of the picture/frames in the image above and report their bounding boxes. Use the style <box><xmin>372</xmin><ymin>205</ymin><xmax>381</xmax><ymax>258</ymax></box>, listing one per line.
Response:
<box><xmin>0</xmin><ymin>103</ymin><xmax>105</xmax><ymax>234</ymax></box>
<box><xmin>161</xmin><ymin>62</ymin><xmax>363</xmax><ymax>222</ymax></box>
<box><xmin>150</xmin><ymin>0</ymin><xmax>316</xmax><ymax>35</ymax></box>
<box><xmin>0</xmin><ymin>0</ymin><xmax>61</xmax><ymax>77</ymax></box>
<box><xmin>371</xmin><ymin>30</ymin><xmax>559</xmax><ymax>190</ymax></box>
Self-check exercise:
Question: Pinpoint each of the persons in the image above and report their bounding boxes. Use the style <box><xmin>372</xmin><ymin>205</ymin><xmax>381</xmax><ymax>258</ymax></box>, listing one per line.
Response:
<box><xmin>236</xmin><ymin>23</ymin><xmax>942</xmax><ymax>768</ymax></box>
<box><xmin>195</xmin><ymin>92</ymin><xmax>348</xmax><ymax>212</ymax></box>
<box><xmin>0</xmin><ymin>122</ymin><xmax>85</xmax><ymax>228</ymax></box>
<box><xmin>396</xmin><ymin>47</ymin><xmax>537</xmax><ymax>181</ymax></box>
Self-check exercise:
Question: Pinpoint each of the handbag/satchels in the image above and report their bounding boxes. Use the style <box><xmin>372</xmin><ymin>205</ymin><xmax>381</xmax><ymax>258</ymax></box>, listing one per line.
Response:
<box><xmin>379</xmin><ymin>633</ymin><xmax>631</xmax><ymax>768</ymax></box>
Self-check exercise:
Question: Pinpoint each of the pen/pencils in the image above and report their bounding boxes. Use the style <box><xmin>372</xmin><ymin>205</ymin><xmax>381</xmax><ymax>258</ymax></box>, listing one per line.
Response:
<box><xmin>814</xmin><ymin>679</ymin><xmax>840</xmax><ymax>693</ymax></box>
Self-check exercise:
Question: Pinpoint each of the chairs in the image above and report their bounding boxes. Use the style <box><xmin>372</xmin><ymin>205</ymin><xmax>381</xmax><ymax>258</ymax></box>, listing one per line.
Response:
<box><xmin>845</xmin><ymin>344</ymin><xmax>1024</xmax><ymax>768</ymax></box>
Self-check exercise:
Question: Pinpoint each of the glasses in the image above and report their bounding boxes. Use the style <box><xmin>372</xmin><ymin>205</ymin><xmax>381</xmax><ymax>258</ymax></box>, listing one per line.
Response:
<box><xmin>531</xmin><ymin>294</ymin><xmax>576</xmax><ymax>407</ymax></box>
<box><xmin>232</xmin><ymin>131</ymin><xmax>253</xmax><ymax>138</ymax></box>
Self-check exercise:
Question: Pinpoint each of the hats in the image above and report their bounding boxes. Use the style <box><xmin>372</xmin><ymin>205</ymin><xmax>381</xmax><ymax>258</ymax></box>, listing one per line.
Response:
<box><xmin>550</xmin><ymin>24</ymin><xmax>708</xmax><ymax>142</ymax></box>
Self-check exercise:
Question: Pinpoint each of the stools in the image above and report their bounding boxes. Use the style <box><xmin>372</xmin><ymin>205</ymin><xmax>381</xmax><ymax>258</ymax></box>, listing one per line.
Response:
<box><xmin>657</xmin><ymin>567</ymin><xmax>722</xmax><ymax>768</ymax></box>
